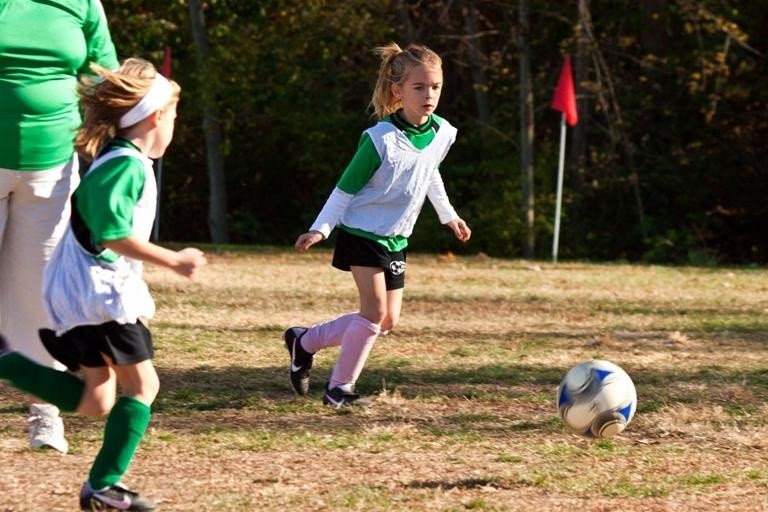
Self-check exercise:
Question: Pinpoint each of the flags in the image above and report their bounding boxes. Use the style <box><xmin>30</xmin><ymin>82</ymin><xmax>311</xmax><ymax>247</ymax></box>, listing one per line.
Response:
<box><xmin>162</xmin><ymin>48</ymin><xmax>172</xmax><ymax>78</ymax></box>
<box><xmin>551</xmin><ymin>58</ymin><xmax>579</xmax><ymax>127</ymax></box>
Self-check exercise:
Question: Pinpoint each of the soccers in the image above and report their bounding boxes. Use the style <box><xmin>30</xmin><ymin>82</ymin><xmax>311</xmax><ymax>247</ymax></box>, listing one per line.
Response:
<box><xmin>556</xmin><ymin>356</ymin><xmax>639</xmax><ymax>440</ymax></box>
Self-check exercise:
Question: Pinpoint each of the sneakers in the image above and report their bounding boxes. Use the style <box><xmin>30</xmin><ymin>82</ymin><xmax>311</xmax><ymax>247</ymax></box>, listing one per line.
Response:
<box><xmin>81</xmin><ymin>480</ymin><xmax>156</xmax><ymax>512</ymax></box>
<box><xmin>283</xmin><ymin>326</ymin><xmax>314</xmax><ymax>398</ymax></box>
<box><xmin>323</xmin><ymin>379</ymin><xmax>360</xmax><ymax>409</ymax></box>
<box><xmin>27</xmin><ymin>404</ymin><xmax>68</xmax><ymax>454</ymax></box>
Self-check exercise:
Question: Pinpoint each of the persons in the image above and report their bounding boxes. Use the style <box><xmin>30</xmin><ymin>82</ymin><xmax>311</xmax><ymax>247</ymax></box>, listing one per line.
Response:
<box><xmin>1</xmin><ymin>1</ymin><xmax>121</xmax><ymax>455</ymax></box>
<box><xmin>0</xmin><ymin>57</ymin><xmax>208</xmax><ymax>511</ymax></box>
<box><xmin>284</xmin><ymin>43</ymin><xmax>473</xmax><ymax>405</ymax></box>
